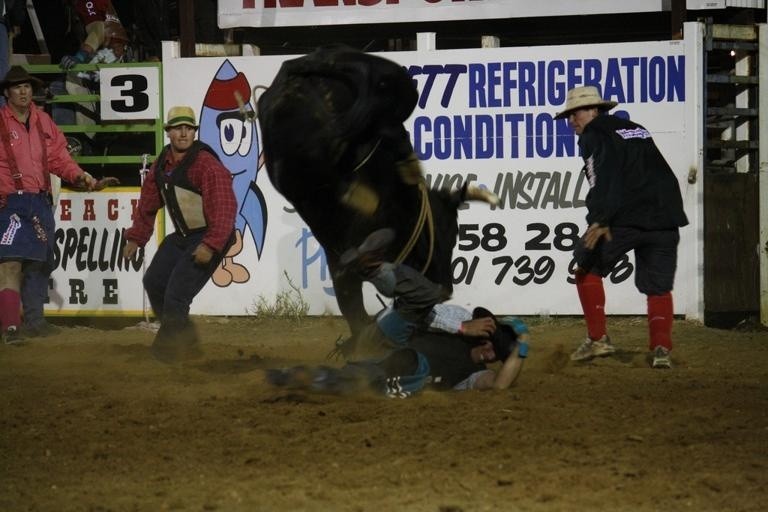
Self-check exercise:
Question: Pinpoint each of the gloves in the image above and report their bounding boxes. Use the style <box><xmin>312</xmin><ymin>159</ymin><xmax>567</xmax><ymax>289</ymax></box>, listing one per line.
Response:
<box><xmin>61</xmin><ymin>44</ymin><xmax>93</xmax><ymax>70</ymax></box>
<box><xmin>499</xmin><ymin>316</ymin><xmax>530</xmax><ymax>336</ymax></box>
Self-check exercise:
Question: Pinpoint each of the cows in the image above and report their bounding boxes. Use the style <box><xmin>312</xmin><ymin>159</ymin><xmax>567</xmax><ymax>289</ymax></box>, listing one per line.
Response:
<box><xmin>258</xmin><ymin>42</ymin><xmax>488</xmax><ymax>338</ymax></box>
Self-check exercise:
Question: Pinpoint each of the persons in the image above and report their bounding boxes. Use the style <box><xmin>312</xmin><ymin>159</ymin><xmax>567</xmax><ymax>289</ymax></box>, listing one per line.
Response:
<box><xmin>0</xmin><ymin>0</ymin><xmax>168</xmax><ymax>193</ymax></box>
<box><xmin>0</xmin><ymin>62</ymin><xmax>100</xmax><ymax>352</ymax></box>
<box><xmin>553</xmin><ymin>86</ymin><xmax>690</xmax><ymax>371</ymax></box>
<box><xmin>117</xmin><ymin>103</ymin><xmax>238</xmax><ymax>371</ymax></box>
<box><xmin>259</xmin><ymin>221</ymin><xmax>535</xmax><ymax>399</ymax></box>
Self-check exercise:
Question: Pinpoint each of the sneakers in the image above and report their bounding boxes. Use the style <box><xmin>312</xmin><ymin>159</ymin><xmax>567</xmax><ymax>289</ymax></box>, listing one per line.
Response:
<box><xmin>650</xmin><ymin>345</ymin><xmax>671</xmax><ymax>367</ymax></box>
<box><xmin>23</xmin><ymin>321</ymin><xmax>61</xmax><ymax>337</ymax></box>
<box><xmin>1</xmin><ymin>325</ymin><xmax>24</xmax><ymax>346</ymax></box>
<box><xmin>148</xmin><ymin>345</ymin><xmax>205</xmax><ymax>368</ymax></box>
<box><xmin>569</xmin><ymin>333</ymin><xmax>615</xmax><ymax>361</ymax></box>
<box><xmin>339</xmin><ymin>227</ymin><xmax>396</xmax><ymax>280</ymax></box>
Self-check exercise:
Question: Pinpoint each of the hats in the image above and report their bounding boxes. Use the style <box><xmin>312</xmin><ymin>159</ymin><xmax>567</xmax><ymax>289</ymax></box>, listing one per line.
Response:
<box><xmin>0</xmin><ymin>65</ymin><xmax>43</xmax><ymax>96</ymax></box>
<box><xmin>162</xmin><ymin>106</ymin><xmax>200</xmax><ymax>132</ymax></box>
<box><xmin>552</xmin><ymin>86</ymin><xmax>619</xmax><ymax>120</ymax></box>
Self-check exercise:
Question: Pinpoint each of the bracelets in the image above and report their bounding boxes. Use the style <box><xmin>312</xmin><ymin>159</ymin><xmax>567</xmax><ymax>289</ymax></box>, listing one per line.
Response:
<box><xmin>457</xmin><ymin>322</ymin><xmax>465</xmax><ymax>334</ymax></box>
